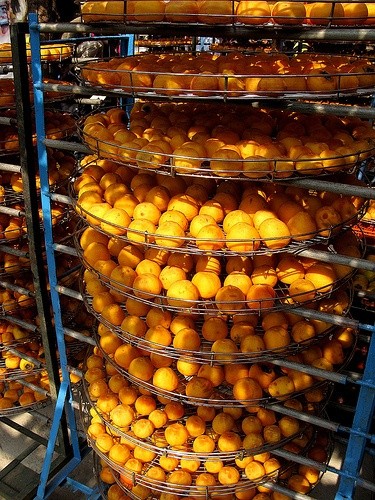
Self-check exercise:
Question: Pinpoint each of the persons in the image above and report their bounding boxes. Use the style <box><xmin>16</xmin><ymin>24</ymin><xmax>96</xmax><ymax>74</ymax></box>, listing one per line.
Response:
<box><xmin>10</xmin><ymin>0</ymin><xmax>120</xmax><ymax>121</ymax></box>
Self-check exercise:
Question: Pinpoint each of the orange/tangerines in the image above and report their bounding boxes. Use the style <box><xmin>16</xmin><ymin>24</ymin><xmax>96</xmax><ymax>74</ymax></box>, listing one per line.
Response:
<box><xmin>0</xmin><ymin>0</ymin><xmax>375</xmax><ymax>500</ymax></box>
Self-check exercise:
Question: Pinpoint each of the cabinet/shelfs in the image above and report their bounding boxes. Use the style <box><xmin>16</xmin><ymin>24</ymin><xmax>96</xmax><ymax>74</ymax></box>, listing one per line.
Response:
<box><xmin>0</xmin><ymin>0</ymin><xmax>375</xmax><ymax>499</ymax></box>
